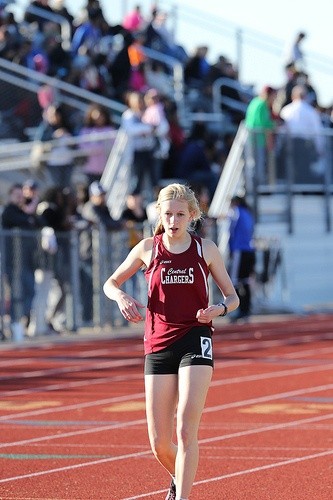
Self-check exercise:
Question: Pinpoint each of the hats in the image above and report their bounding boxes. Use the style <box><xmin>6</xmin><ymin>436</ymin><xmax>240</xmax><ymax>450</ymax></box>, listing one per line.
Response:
<box><xmin>89</xmin><ymin>181</ymin><xmax>106</xmax><ymax>196</ymax></box>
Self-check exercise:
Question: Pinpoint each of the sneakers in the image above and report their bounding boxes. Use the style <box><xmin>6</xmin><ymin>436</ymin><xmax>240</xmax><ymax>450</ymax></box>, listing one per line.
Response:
<box><xmin>165</xmin><ymin>476</ymin><xmax>176</xmax><ymax>500</ymax></box>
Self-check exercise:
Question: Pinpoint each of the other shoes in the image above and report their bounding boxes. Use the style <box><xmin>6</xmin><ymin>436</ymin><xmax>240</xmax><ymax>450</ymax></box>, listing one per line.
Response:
<box><xmin>12</xmin><ymin>322</ymin><xmax>23</xmax><ymax>342</ymax></box>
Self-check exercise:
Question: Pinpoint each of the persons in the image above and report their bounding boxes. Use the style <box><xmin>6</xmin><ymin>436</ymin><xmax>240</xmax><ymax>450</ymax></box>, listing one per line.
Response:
<box><xmin>102</xmin><ymin>183</ymin><xmax>240</xmax><ymax>500</ymax></box>
<box><xmin>0</xmin><ymin>0</ymin><xmax>333</xmax><ymax>341</ymax></box>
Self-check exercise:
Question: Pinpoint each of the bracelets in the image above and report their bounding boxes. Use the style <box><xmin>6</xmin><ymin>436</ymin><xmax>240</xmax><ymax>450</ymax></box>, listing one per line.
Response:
<box><xmin>216</xmin><ymin>303</ymin><xmax>228</xmax><ymax>317</ymax></box>
